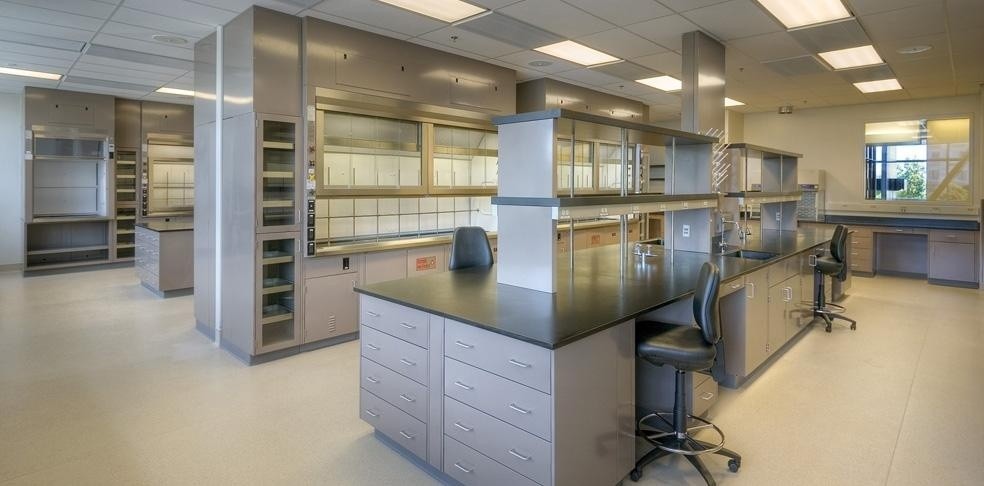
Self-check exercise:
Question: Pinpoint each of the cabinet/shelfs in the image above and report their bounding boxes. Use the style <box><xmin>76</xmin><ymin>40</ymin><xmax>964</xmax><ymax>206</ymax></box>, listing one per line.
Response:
<box><xmin>821</xmin><ymin>197</ymin><xmax>984</xmax><ymax>289</ymax></box>
<box><xmin>17</xmin><ymin>85</ymin><xmax>113</xmax><ymax>277</ymax></box>
<box><xmin>306</xmin><ymin>107</ymin><xmax>807</xmax><ymax>343</ymax></box>
<box><xmin>194</xmin><ymin>111</ymin><xmax>304</xmax><ymax>366</ymax></box>
<box><xmin>352</xmin><ymin>220</ymin><xmax>855</xmax><ymax>484</ymax></box>
<box><xmin>112</xmin><ymin>97</ymin><xmax>141</xmax><ymax>269</ymax></box>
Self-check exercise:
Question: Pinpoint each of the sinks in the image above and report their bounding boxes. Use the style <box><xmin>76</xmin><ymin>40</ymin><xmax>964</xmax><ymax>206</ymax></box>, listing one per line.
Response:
<box><xmin>720</xmin><ymin>248</ymin><xmax>782</xmax><ymax>261</ymax></box>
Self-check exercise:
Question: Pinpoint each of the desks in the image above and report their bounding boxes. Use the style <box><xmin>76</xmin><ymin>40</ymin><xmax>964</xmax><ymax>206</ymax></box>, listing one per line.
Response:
<box><xmin>133</xmin><ymin>221</ymin><xmax>193</xmax><ymax>299</ymax></box>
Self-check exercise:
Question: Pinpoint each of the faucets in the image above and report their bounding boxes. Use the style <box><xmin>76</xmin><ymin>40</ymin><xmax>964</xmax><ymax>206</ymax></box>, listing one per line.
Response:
<box><xmin>717</xmin><ymin>217</ymin><xmax>744</xmax><ymax>253</ymax></box>
